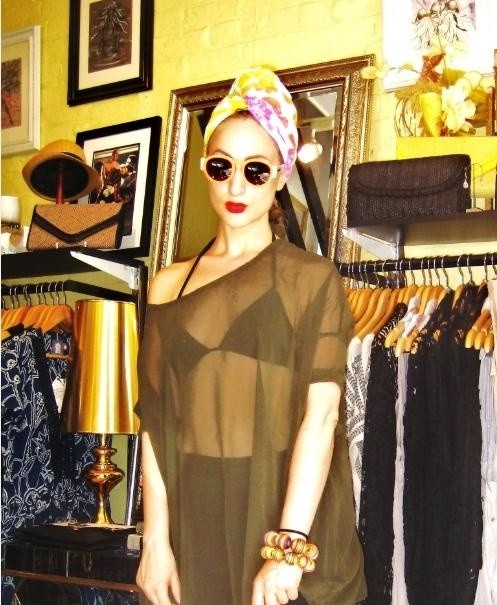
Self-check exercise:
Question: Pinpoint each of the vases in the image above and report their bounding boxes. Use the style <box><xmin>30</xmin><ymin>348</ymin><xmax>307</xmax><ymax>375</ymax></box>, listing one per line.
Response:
<box><xmin>397</xmin><ymin>136</ymin><xmax>497</xmax><ymax>200</ymax></box>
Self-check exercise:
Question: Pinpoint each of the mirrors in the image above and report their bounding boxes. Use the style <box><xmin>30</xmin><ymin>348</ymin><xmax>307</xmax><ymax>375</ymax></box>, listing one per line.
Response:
<box><xmin>149</xmin><ymin>51</ymin><xmax>378</xmax><ymax>289</ymax></box>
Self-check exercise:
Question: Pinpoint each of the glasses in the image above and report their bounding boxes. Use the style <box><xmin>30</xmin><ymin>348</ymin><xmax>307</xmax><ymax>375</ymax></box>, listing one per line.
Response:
<box><xmin>200</xmin><ymin>154</ymin><xmax>284</xmax><ymax>186</ymax></box>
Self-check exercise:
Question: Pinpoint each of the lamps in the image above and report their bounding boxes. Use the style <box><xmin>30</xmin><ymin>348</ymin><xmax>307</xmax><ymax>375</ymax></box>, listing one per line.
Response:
<box><xmin>297</xmin><ymin>115</ymin><xmax>335</xmax><ymax>164</ymax></box>
<box><xmin>57</xmin><ymin>297</ymin><xmax>142</xmax><ymax>531</ymax></box>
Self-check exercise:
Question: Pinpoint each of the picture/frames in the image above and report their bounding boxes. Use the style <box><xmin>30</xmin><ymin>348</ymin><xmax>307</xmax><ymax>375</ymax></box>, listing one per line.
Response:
<box><xmin>1</xmin><ymin>24</ymin><xmax>41</xmax><ymax>156</ymax></box>
<box><xmin>73</xmin><ymin>115</ymin><xmax>162</xmax><ymax>259</ymax></box>
<box><xmin>67</xmin><ymin>0</ymin><xmax>154</xmax><ymax>107</ymax></box>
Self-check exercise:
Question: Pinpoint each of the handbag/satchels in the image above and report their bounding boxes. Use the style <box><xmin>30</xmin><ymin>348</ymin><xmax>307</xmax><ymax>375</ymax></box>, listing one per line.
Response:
<box><xmin>346</xmin><ymin>136</ymin><xmax>497</xmax><ymax>228</ymax></box>
<box><xmin>26</xmin><ymin>202</ymin><xmax>124</xmax><ymax>251</ymax></box>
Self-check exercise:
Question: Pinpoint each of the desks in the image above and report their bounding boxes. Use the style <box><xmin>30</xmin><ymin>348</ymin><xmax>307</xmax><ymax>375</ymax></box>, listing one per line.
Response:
<box><xmin>2</xmin><ymin>540</ymin><xmax>152</xmax><ymax>605</ymax></box>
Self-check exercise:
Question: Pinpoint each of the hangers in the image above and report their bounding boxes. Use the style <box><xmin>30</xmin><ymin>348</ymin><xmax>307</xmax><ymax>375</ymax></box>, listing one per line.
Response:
<box><xmin>333</xmin><ymin>251</ymin><xmax>496</xmax><ymax>356</ymax></box>
<box><xmin>1</xmin><ymin>281</ymin><xmax>75</xmax><ymax>365</ymax></box>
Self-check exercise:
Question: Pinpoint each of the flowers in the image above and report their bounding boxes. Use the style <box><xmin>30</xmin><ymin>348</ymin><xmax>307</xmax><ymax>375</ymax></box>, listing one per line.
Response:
<box><xmin>358</xmin><ymin>7</ymin><xmax>495</xmax><ymax>137</ymax></box>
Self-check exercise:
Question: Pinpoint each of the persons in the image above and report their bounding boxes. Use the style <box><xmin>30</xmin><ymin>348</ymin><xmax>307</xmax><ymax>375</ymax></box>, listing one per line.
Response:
<box><xmin>133</xmin><ymin>62</ymin><xmax>373</xmax><ymax>605</ymax></box>
<box><xmin>90</xmin><ymin>149</ymin><xmax>136</xmax><ymax>205</ymax></box>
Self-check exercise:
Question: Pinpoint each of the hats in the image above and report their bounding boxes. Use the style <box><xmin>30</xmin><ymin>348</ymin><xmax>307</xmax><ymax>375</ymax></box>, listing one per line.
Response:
<box><xmin>22</xmin><ymin>141</ymin><xmax>99</xmax><ymax>201</ymax></box>
<box><xmin>125</xmin><ymin>155</ymin><xmax>136</xmax><ymax>172</ymax></box>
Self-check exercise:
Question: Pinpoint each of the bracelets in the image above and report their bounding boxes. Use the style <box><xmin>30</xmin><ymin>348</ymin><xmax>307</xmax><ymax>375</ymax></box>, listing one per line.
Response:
<box><xmin>278</xmin><ymin>529</ymin><xmax>309</xmax><ymax>540</ymax></box>
<box><xmin>263</xmin><ymin>531</ymin><xmax>318</xmax><ymax>560</ymax></box>
<box><xmin>260</xmin><ymin>546</ymin><xmax>315</xmax><ymax>575</ymax></box>
